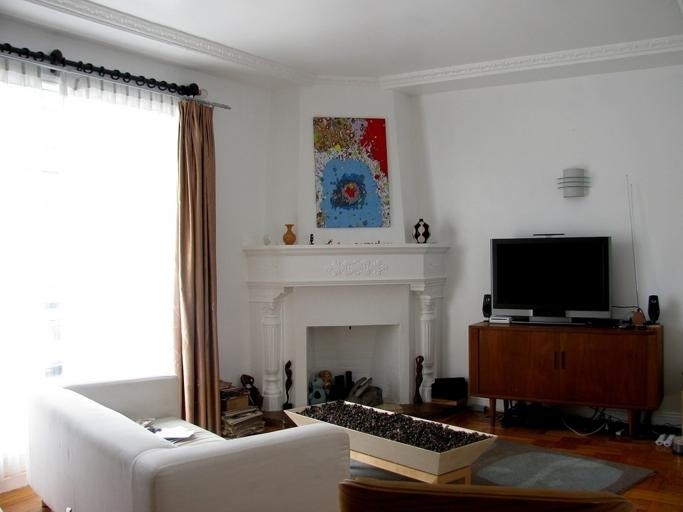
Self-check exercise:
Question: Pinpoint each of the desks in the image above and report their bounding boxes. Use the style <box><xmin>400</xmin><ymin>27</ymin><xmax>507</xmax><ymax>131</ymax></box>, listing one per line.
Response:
<box><xmin>350</xmin><ymin>450</ymin><xmax>473</xmax><ymax>487</ymax></box>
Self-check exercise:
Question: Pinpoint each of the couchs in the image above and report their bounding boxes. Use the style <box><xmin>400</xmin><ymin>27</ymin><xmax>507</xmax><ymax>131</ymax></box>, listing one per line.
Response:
<box><xmin>25</xmin><ymin>368</ymin><xmax>351</xmax><ymax>512</ymax></box>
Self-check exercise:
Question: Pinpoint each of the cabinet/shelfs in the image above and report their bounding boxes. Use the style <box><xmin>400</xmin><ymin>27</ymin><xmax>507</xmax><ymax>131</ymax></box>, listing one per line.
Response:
<box><xmin>463</xmin><ymin>318</ymin><xmax>664</xmax><ymax>436</ymax></box>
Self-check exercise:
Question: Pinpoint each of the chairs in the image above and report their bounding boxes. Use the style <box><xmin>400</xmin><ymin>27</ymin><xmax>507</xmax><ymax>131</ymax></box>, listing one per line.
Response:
<box><xmin>332</xmin><ymin>475</ymin><xmax>633</xmax><ymax>512</ymax></box>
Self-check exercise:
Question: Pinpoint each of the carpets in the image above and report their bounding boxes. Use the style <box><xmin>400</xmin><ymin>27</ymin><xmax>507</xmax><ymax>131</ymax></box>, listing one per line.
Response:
<box><xmin>350</xmin><ymin>414</ymin><xmax>659</xmax><ymax>494</ymax></box>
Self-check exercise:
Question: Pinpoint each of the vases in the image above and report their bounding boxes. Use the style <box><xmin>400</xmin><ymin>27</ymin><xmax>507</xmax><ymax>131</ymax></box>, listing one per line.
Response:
<box><xmin>282</xmin><ymin>223</ymin><xmax>297</xmax><ymax>245</ymax></box>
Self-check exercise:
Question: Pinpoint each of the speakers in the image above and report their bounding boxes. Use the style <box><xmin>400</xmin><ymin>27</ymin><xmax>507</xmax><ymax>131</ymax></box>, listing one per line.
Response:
<box><xmin>482</xmin><ymin>294</ymin><xmax>492</xmax><ymax>318</ymax></box>
<box><xmin>648</xmin><ymin>295</ymin><xmax>661</xmax><ymax>325</ymax></box>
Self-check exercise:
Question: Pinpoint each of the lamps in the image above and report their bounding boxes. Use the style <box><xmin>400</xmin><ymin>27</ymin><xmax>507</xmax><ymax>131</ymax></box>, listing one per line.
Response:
<box><xmin>556</xmin><ymin>167</ymin><xmax>592</xmax><ymax>200</ymax></box>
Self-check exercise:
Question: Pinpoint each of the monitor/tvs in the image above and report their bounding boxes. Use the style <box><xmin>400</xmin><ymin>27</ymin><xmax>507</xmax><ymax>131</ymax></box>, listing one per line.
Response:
<box><xmin>489</xmin><ymin>233</ymin><xmax>611</xmax><ymax>325</ymax></box>
